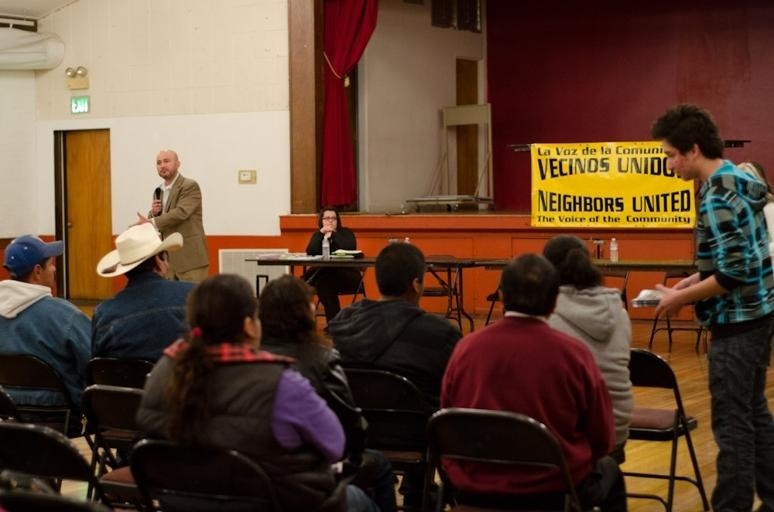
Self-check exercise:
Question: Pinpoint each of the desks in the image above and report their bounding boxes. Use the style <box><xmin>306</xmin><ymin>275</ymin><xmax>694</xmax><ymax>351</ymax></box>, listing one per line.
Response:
<box><xmin>245</xmin><ymin>257</ymin><xmax>496</xmax><ymax>333</ymax></box>
<box><xmin>470</xmin><ymin>259</ymin><xmax>694</xmax><ymax>270</ymax></box>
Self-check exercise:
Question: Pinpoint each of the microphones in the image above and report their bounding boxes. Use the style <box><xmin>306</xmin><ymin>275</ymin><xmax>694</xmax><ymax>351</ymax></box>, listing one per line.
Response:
<box><xmin>155</xmin><ymin>187</ymin><xmax>161</xmax><ymax>216</ymax></box>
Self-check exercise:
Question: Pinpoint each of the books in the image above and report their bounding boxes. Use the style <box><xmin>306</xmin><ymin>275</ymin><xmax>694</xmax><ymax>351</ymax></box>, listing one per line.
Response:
<box><xmin>332</xmin><ymin>249</ymin><xmax>364</xmax><ymax>262</ymax></box>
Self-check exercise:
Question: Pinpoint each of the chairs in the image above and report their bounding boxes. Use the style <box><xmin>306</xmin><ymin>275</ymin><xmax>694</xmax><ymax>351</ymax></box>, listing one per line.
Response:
<box><xmin>484</xmin><ymin>288</ymin><xmax>501</xmax><ymax>325</ymax></box>
<box><xmin>421</xmin><ymin>255</ymin><xmax>462</xmax><ymax>331</ymax></box>
<box><xmin>649</xmin><ymin>270</ymin><xmax>710</xmax><ymax>352</ymax></box>
<box><xmin>603</xmin><ymin>269</ymin><xmax>630</xmax><ymax>311</ymax></box>
<box><xmin>316</xmin><ymin>267</ymin><xmax>368</xmax><ymax>318</ymax></box>
<box><xmin>0</xmin><ymin>348</ymin><xmax>711</xmax><ymax>512</ymax></box>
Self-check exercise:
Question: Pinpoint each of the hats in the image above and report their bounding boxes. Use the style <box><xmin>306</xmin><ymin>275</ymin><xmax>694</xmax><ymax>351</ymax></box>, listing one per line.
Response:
<box><xmin>96</xmin><ymin>222</ymin><xmax>184</xmax><ymax>278</ymax></box>
<box><xmin>4</xmin><ymin>234</ymin><xmax>65</xmax><ymax>276</ymax></box>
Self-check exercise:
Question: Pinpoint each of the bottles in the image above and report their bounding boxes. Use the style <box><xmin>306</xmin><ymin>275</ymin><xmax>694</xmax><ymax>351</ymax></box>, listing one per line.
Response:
<box><xmin>609</xmin><ymin>238</ymin><xmax>618</xmax><ymax>262</ymax></box>
<box><xmin>404</xmin><ymin>237</ymin><xmax>411</xmax><ymax>244</ymax></box>
<box><xmin>322</xmin><ymin>236</ymin><xmax>330</xmax><ymax>260</ymax></box>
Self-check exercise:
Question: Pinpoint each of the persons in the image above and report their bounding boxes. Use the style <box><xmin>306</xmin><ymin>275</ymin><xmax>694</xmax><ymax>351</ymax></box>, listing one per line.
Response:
<box><xmin>136</xmin><ymin>272</ymin><xmax>376</xmax><ymax>512</ymax></box>
<box><xmin>536</xmin><ymin>237</ymin><xmax>633</xmax><ymax>456</ymax></box>
<box><xmin>91</xmin><ymin>222</ymin><xmax>196</xmax><ymax>463</ymax></box>
<box><xmin>298</xmin><ymin>207</ymin><xmax>361</xmax><ymax>333</ymax></box>
<box><xmin>649</xmin><ymin>105</ymin><xmax>774</xmax><ymax>512</ymax></box>
<box><xmin>0</xmin><ymin>235</ymin><xmax>93</xmax><ymax>437</ymax></box>
<box><xmin>322</xmin><ymin>241</ymin><xmax>464</xmax><ymax>511</ymax></box>
<box><xmin>258</xmin><ymin>272</ymin><xmax>398</xmax><ymax>512</ymax></box>
<box><xmin>128</xmin><ymin>149</ymin><xmax>210</xmax><ymax>285</ymax></box>
<box><xmin>439</xmin><ymin>252</ymin><xmax>628</xmax><ymax>510</ymax></box>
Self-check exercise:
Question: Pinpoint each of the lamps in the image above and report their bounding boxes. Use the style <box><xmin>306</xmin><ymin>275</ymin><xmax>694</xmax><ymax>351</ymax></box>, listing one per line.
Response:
<box><xmin>65</xmin><ymin>65</ymin><xmax>89</xmax><ymax>90</ymax></box>
